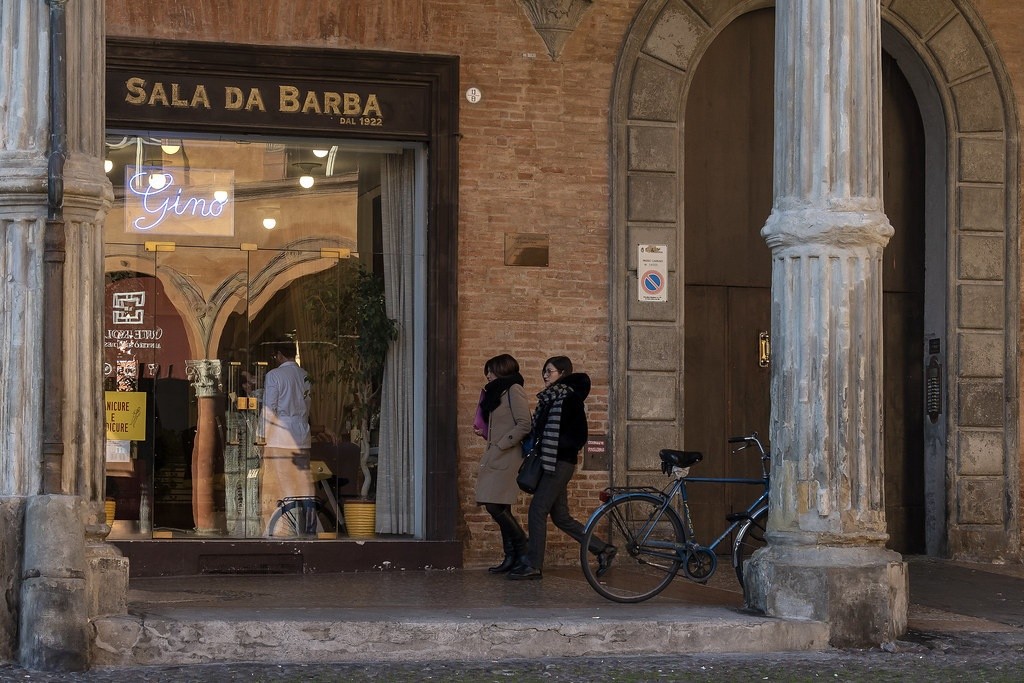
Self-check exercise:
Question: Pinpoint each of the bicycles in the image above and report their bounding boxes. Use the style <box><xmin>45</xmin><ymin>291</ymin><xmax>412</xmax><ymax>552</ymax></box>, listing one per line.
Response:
<box><xmin>267</xmin><ymin>474</ymin><xmax>349</xmax><ymax>539</ymax></box>
<box><xmin>580</xmin><ymin>429</ymin><xmax>773</xmax><ymax>605</ymax></box>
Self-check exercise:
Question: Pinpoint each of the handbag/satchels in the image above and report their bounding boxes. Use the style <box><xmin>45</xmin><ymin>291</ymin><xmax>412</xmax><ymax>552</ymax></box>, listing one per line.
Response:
<box><xmin>516</xmin><ymin>449</ymin><xmax>545</xmax><ymax>494</ymax></box>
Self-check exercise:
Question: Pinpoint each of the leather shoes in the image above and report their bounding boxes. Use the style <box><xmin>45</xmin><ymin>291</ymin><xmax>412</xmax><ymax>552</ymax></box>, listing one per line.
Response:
<box><xmin>508</xmin><ymin>565</ymin><xmax>543</xmax><ymax>579</ymax></box>
<box><xmin>595</xmin><ymin>544</ymin><xmax>618</xmax><ymax>577</ymax></box>
<box><xmin>511</xmin><ymin>539</ymin><xmax>530</xmax><ymax>571</ymax></box>
<box><xmin>489</xmin><ymin>555</ymin><xmax>517</xmax><ymax>573</ymax></box>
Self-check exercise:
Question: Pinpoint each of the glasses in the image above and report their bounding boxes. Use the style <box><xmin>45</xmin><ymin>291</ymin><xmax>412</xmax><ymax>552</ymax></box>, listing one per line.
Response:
<box><xmin>543</xmin><ymin>369</ymin><xmax>558</xmax><ymax>376</ymax></box>
<box><xmin>272</xmin><ymin>352</ymin><xmax>277</xmax><ymax>359</ymax></box>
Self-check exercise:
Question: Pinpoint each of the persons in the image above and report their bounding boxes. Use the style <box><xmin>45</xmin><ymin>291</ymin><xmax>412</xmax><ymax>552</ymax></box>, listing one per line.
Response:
<box><xmin>506</xmin><ymin>356</ymin><xmax>617</xmax><ymax>580</ymax></box>
<box><xmin>474</xmin><ymin>354</ymin><xmax>528</xmax><ymax>573</ymax></box>
<box><xmin>238</xmin><ymin>338</ymin><xmax>326</xmax><ymax>537</ymax></box>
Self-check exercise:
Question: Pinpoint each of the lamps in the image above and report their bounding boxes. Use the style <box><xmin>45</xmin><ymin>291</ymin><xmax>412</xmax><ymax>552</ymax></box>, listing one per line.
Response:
<box><xmin>161</xmin><ymin>146</ymin><xmax>180</xmax><ymax>155</ymax></box>
<box><xmin>312</xmin><ymin>150</ymin><xmax>328</xmax><ymax>158</ymax></box>
<box><xmin>104</xmin><ymin>146</ymin><xmax>113</xmax><ymax>173</ymax></box>
<box><xmin>209</xmin><ymin>178</ymin><xmax>236</xmax><ymax>203</ymax></box>
<box><xmin>292</xmin><ymin>163</ymin><xmax>322</xmax><ymax>188</ymax></box>
<box><xmin>257</xmin><ymin>207</ymin><xmax>281</xmax><ymax>230</ymax></box>
<box><xmin>146</xmin><ymin>160</ymin><xmax>172</xmax><ymax>190</ymax></box>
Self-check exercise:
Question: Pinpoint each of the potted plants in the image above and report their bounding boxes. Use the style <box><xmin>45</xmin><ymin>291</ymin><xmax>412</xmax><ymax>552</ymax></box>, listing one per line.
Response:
<box><xmin>302</xmin><ymin>255</ymin><xmax>402</xmax><ymax>540</ymax></box>
